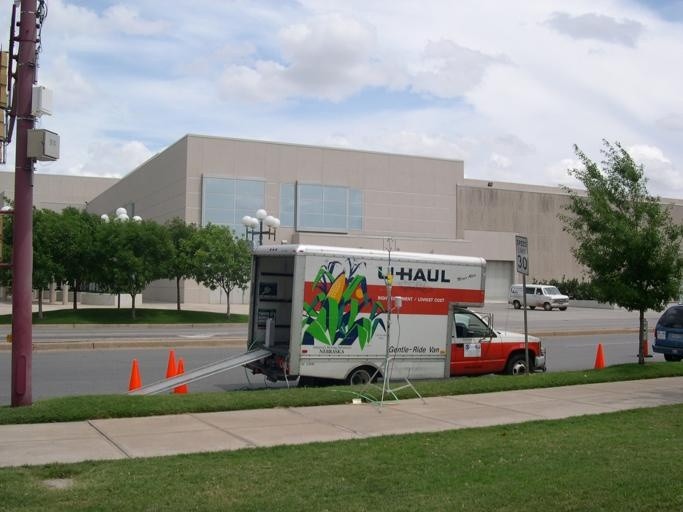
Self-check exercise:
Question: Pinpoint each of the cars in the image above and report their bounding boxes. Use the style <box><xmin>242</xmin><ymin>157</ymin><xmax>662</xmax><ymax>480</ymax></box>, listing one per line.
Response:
<box><xmin>652</xmin><ymin>304</ymin><xmax>683</xmax><ymax>362</ymax></box>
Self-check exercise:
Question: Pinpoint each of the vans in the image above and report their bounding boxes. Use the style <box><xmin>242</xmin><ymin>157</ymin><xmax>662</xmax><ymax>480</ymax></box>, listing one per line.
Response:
<box><xmin>508</xmin><ymin>283</ymin><xmax>569</xmax><ymax>310</ymax></box>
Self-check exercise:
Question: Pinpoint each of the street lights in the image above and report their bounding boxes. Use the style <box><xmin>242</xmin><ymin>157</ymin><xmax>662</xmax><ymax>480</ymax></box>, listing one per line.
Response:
<box><xmin>100</xmin><ymin>208</ymin><xmax>143</xmax><ymax>226</ymax></box>
<box><xmin>241</xmin><ymin>209</ymin><xmax>280</xmax><ymax>246</ymax></box>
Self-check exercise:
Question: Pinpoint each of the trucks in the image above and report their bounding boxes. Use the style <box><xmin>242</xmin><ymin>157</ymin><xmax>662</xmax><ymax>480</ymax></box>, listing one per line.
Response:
<box><xmin>243</xmin><ymin>243</ymin><xmax>546</xmax><ymax>386</ymax></box>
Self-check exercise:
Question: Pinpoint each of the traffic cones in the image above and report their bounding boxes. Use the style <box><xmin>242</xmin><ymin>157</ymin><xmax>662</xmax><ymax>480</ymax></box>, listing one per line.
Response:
<box><xmin>127</xmin><ymin>358</ymin><xmax>142</xmax><ymax>391</ymax></box>
<box><xmin>593</xmin><ymin>343</ymin><xmax>606</xmax><ymax>368</ymax></box>
<box><xmin>166</xmin><ymin>351</ymin><xmax>188</xmax><ymax>394</ymax></box>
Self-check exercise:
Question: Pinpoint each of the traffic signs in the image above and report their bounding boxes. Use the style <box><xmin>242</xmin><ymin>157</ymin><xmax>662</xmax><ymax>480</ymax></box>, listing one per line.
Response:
<box><xmin>516</xmin><ymin>236</ymin><xmax>529</xmax><ymax>275</ymax></box>
